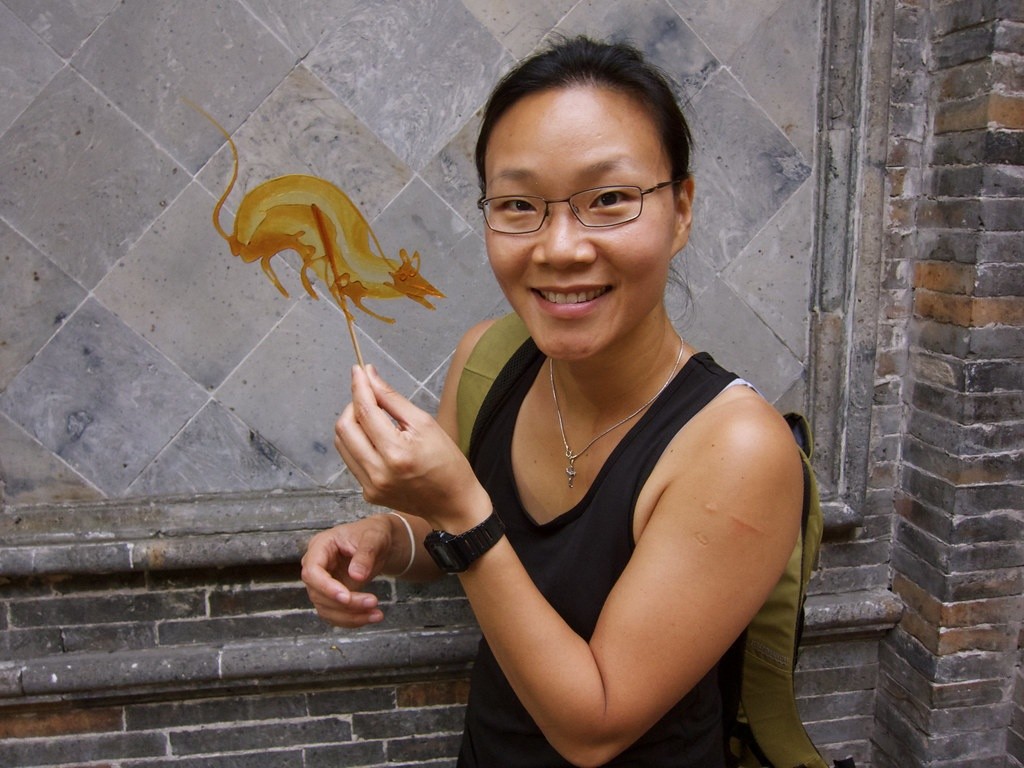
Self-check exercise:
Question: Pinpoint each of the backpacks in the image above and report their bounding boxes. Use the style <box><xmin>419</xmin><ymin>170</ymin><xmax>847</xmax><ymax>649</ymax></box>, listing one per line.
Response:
<box><xmin>455</xmin><ymin>310</ymin><xmax>831</xmax><ymax>768</ymax></box>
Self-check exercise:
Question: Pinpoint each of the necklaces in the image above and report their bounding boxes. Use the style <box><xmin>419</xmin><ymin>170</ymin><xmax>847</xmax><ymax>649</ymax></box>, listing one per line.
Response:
<box><xmin>549</xmin><ymin>334</ymin><xmax>684</xmax><ymax>489</ymax></box>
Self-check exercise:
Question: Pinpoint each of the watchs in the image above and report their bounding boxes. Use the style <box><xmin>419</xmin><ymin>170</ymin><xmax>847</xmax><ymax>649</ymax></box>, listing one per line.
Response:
<box><xmin>424</xmin><ymin>509</ymin><xmax>506</xmax><ymax>573</ymax></box>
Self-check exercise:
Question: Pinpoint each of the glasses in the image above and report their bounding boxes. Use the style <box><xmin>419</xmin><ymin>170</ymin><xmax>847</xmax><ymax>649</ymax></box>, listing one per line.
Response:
<box><xmin>477</xmin><ymin>179</ymin><xmax>681</xmax><ymax>235</ymax></box>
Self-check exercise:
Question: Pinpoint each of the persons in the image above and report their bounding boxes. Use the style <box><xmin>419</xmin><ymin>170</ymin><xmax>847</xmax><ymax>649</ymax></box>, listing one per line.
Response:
<box><xmin>302</xmin><ymin>33</ymin><xmax>823</xmax><ymax>768</ymax></box>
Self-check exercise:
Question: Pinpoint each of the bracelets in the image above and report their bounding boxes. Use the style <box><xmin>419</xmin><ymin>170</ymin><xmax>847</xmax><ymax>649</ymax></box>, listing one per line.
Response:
<box><xmin>386</xmin><ymin>513</ymin><xmax>416</xmax><ymax>579</ymax></box>
